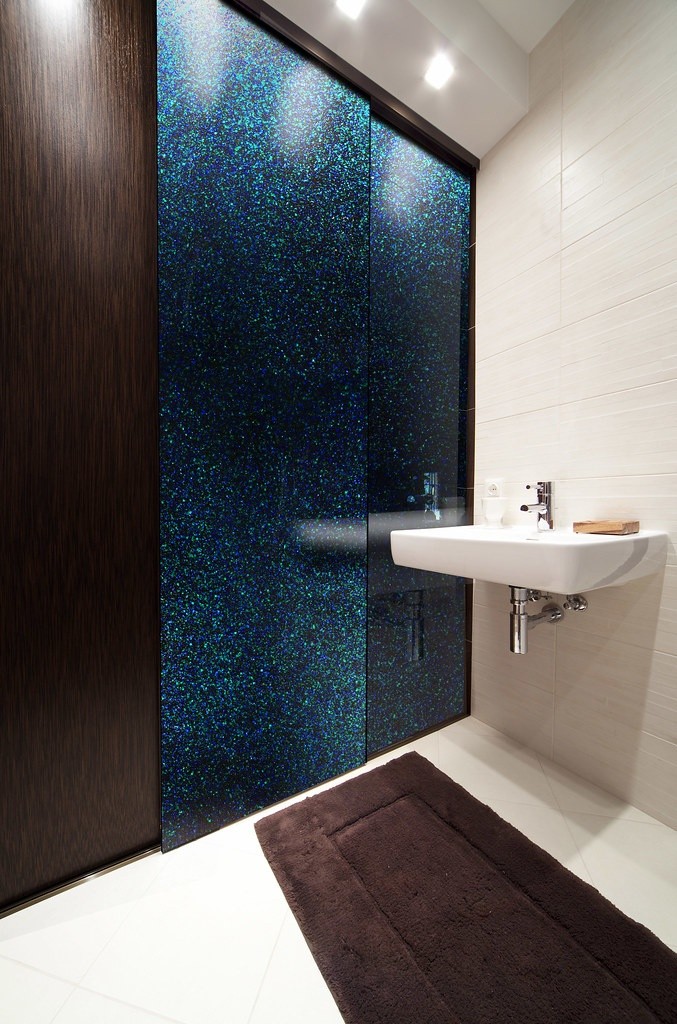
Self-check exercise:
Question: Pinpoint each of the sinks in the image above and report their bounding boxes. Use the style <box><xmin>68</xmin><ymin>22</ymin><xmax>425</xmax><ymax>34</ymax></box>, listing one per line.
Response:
<box><xmin>283</xmin><ymin>506</ymin><xmax>467</xmax><ymax>565</ymax></box>
<box><xmin>390</xmin><ymin>523</ymin><xmax>669</xmax><ymax>596</ymax></box>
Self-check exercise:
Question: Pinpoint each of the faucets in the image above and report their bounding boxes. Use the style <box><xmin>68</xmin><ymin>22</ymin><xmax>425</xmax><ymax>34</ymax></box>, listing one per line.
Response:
<box><xmin>406</xmin><ymin>471</ymin><xmax>445</xmax><ymax>523</ymax></box>
<box><xmin>519</xmin><ymin>480</ymin><xmax>556</xmax><ymax>532</ymax></box>
